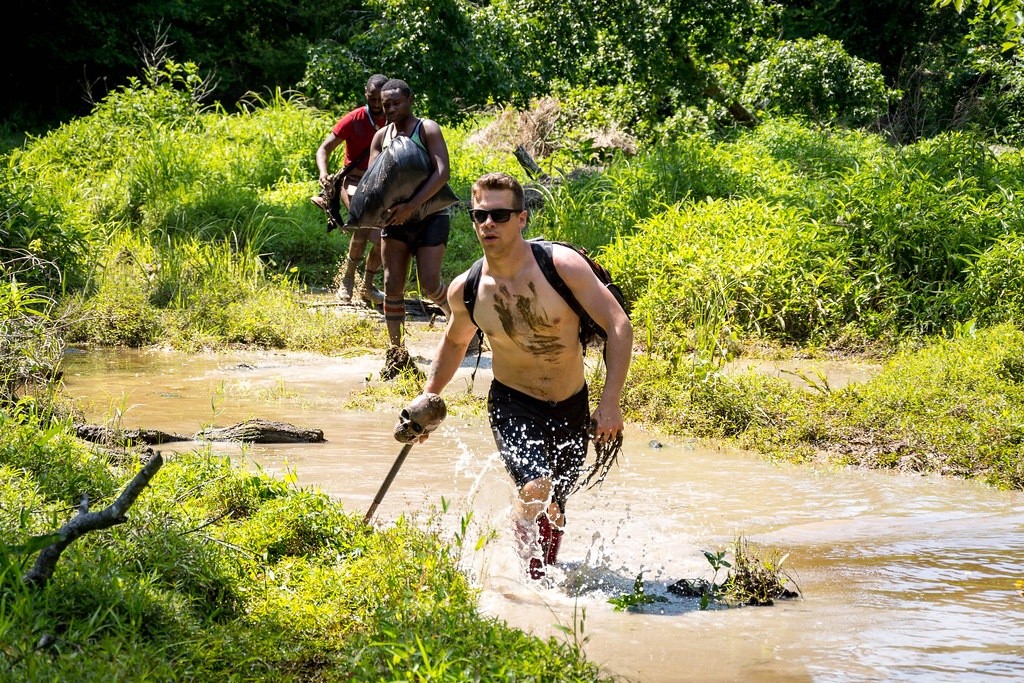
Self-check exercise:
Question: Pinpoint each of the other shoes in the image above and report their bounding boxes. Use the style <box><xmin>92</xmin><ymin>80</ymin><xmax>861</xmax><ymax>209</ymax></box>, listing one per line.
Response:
<box><xmin>381</xmin><ymin>353</ymin><xmax>426</xmax><ymax>382</ymax></box>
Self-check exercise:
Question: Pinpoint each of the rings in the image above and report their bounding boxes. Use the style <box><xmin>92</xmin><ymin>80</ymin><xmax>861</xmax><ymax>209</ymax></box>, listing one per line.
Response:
<box><xmin>401</xmin><ymin>222</ymin><xmax>403</xmax><ymax>225</ymax></box>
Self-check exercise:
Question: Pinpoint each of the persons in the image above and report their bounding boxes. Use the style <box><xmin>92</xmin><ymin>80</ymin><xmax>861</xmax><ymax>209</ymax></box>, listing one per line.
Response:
<box><xmin>316</xmin><ymin>74</ymin><xmax>390</xmax><ymax>303</ymax></box>
<box><xmin>415</xmin><ymin>174</ymin><xmax>634</xmax><ymax>587</ymax></box>
<box><xmin>368</xmin><ymin>79</ymin><xmax>482</xmax><ymax>381</ymax></box>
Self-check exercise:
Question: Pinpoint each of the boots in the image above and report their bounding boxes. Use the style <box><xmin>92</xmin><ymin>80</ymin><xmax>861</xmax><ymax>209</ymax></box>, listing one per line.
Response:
<box><xmin>517</xmin><ymin>516</ymin><xmax>565</xmax><ymax>581</ymax></box>
<box><xmin>339</xmin><ymin>257</ymin><xmax>363</xmax><ymax>303</ymax></box>
<box><xmin>360</xmin><ymin>266</ymin><xmax>385</xmax><ymax>306</ymax></box>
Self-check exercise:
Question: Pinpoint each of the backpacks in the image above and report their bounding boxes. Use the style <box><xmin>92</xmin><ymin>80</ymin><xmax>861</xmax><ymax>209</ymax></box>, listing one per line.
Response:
<box><xmin>464</xmin><ymin>238</ymin><xmax>625</xmax><ymax>342</ymax></box>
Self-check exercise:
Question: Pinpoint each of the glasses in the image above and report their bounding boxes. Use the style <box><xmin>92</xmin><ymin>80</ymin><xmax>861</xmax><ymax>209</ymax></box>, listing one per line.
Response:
<box><xmin>468</xmin><ymin>207</ymin><xmax>520</xmax><ymax>223</ymax></box>
